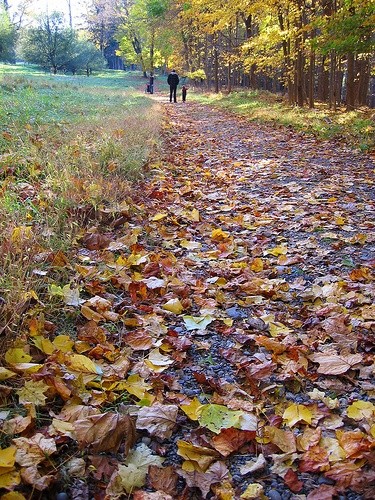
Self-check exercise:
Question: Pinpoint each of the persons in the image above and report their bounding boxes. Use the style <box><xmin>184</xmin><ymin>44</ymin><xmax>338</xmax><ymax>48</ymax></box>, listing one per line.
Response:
<box><xmin>167</xmin><ymin>69</ymin><xmax>180</xmax><ymax>103</ymax></box>
<box><xmin>182</xmin><ymin>86</ymin><xmax>188</xmax><ymax>103</ymax></box>
